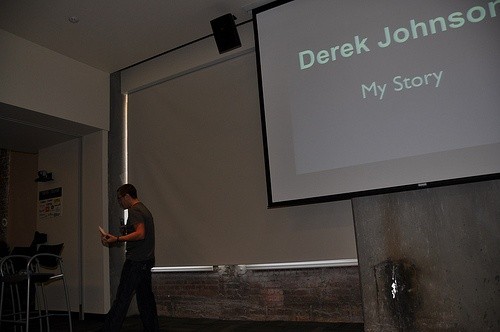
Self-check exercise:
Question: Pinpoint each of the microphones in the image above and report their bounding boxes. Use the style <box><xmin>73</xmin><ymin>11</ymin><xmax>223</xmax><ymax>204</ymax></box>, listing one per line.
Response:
<box><xmin>120</xmin><ymin>218</ymin><xmax>126</xmax><ymax>236</ymax></box>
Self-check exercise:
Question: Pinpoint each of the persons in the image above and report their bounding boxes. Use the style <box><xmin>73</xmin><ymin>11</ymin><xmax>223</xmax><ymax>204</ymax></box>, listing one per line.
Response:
<box><xmin>98</xmin><ymin>184</ymin><xmax>160</xmax><ymax>332</ymax></box>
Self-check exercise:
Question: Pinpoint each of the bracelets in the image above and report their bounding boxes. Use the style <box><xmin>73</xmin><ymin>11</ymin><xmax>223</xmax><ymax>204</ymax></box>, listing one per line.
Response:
<box><xmin>117</xmin><ymin>237</ymin><xmax>119</xmax><ymax>241</ymax></box>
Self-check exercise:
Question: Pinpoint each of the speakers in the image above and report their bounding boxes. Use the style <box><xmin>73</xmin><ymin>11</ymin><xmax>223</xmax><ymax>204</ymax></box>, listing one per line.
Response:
<box><xmin>208</xmin><ymin>13</ymin><xmax>242</xmax><ymax>56</ymax></box>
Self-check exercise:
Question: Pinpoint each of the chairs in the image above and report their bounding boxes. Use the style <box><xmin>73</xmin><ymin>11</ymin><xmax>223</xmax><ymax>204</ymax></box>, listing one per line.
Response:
<box><xmin>1</xmin><ymin>243</ymin><xmax>73</xmax><ymax>332</ymax></box>
